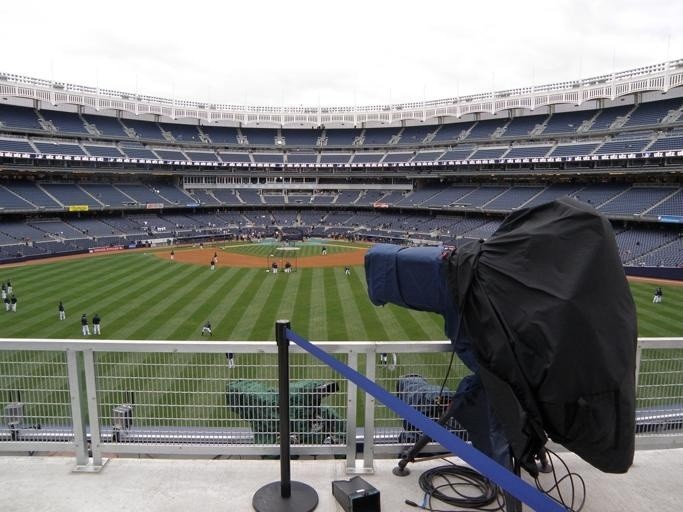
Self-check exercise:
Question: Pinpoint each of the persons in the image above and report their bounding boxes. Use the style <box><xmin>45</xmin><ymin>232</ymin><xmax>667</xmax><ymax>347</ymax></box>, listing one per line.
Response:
<box><xmin>128</xmin><ymin>212</ymin><xmax>453</xmax><ymax>275</ymax></box>
<box><xmin>16</xmin><ymin>229</ymin><xmax>115</xmax><ymax>257</ymax></box>
<box><xmin>93</xmin><ymin>313</ymin><xmax>101</xmax><ymax>335</ymax></box>
<box><xmin>202</xmin><ymin>320</ymin><xmax>214</xmax><ymax>336</ymax></box>
<box><xmin>1</xmin><ymin>278</ymin><xmax>17</xmax><ymax>314</ymax></box>
<box><xmin>653</xmin><ymin>287</ymin><xmax>659</xmax><ymax>303</ymax></box>
<box><xmin>226</xmin><ymin>352</ymin><xmax>236</xmax><ymax>369</ymax></box>
<box><xmin>58</xmin><ymin>301</ymin><xmax>65</xmax><ymax>321</ymax></box>
<box><xmin>381</xmin><ymin>352</ymin><xmax>389</xmax><ymax>364</ymax></box>
<box><xmin>81</xmin><ymin>313</ymin><xmax>91</xmax><ymax>336</ymax></box>
<box><xmin>658</xmin><ymin>288</ymin><xmax>663</xmax><ymax>303</ymax></box>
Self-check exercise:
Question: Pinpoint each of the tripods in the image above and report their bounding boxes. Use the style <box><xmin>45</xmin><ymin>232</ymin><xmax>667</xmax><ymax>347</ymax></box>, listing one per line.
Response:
<box><xmin>392</xmin><ymin>393</ymin><xmax>555</xmax><ymax>512</ymax></box>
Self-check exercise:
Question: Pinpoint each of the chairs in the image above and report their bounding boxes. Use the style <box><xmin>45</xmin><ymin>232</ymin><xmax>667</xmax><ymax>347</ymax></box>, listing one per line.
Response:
<box><xmin>0</xmin><ymin>97</ymin><xmax>683</xmax><ymax>271</ymax></box>
